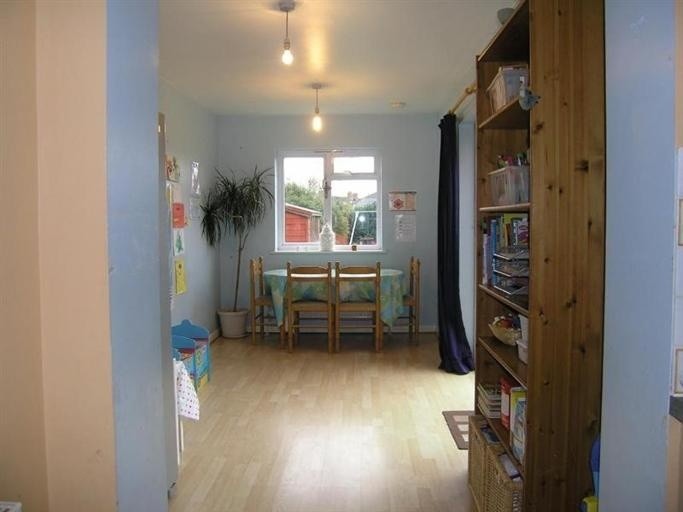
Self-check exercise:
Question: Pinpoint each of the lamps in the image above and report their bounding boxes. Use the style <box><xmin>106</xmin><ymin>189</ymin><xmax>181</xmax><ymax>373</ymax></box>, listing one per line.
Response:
<box><xmin>279</xmin><ymin>0</ymin><xmax>296</xmax><ymax>66</ymax></box>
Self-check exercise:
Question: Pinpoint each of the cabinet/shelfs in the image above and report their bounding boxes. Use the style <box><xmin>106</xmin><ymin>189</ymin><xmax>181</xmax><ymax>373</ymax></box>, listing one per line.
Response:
<box><xmin>469</xmin><ymin>2</ymin><xmax>608</xmax><ymax>512</ymax></box>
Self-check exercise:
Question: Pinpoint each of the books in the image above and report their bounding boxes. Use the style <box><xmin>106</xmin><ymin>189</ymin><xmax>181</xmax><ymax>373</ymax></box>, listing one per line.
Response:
<box><xmin>475</xmin><ymin>211</ymin><xmax>535</xmax><ymax>469</ymax></box>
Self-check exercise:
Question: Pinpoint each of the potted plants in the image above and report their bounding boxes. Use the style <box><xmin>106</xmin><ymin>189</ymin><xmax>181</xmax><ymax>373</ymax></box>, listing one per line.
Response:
<box><xmin>197</xmin><ymin>164</ymin><xmax>276</xmax><ymax>340</ymax></box>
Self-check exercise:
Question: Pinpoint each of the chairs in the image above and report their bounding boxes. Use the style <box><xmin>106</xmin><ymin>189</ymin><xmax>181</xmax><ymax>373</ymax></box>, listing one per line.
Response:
<box><xmin>248</xmin><ymin>255</ymin><xmax>421</xmax><ymax>355</ymax></box>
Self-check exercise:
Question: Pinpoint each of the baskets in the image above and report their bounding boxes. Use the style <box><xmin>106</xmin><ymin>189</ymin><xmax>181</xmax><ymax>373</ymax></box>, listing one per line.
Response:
<box><xmin>468</xmin><ymin>416</ymin><xmax>501</xmax><ymax>512</ymax></box>
<box><xmin>486</xmin><ymin>69</ymin><xmax>529</xmax><ymax>115</ymax></box>
<box><xmin>484</xmin><ymin>444</ymin><xmax>524</xmax><ymax>512</ymax></box>
<box><xmin>488</xmin><ymin>311</ymin><xmax>522</xmax><ymax>346</ymax></box>
<box><xmin>488</xmin><ymin>166</ymin><xmax>530</xmax><ymax>206</ymax></box>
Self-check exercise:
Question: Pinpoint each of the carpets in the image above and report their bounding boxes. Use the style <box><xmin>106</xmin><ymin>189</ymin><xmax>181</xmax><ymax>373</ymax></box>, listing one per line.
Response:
<box><xmin>442</xmin><ymin>410</ymin><xmax>476</xmax><ymax>450</ymax></box>
